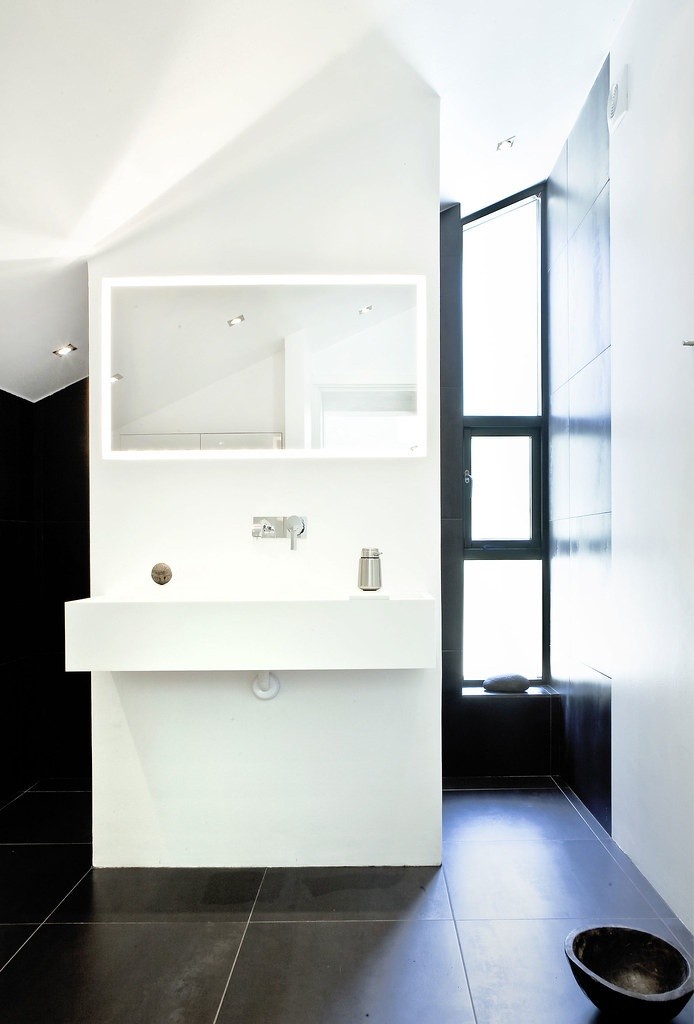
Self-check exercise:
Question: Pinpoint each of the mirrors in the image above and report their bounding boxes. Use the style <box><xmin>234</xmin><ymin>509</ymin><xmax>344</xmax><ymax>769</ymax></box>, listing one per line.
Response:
<box><xmin>96</xmin><ymin>273</ymin><xmax>428</xmax><ymax>461</ymax></box>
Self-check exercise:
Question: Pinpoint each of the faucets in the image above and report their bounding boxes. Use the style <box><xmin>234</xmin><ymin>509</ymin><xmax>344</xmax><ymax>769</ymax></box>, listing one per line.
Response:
<box><xmin>260</xmin><ymin>524</ymin><xmax>267</xmax><ymax>539</ymax></box>
